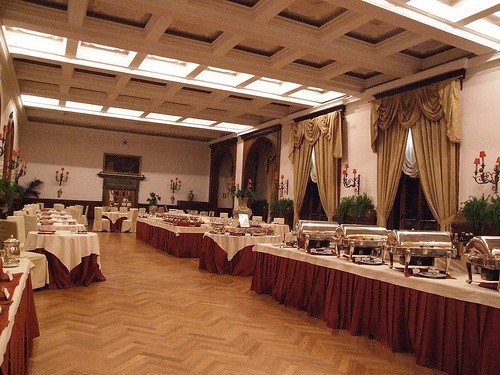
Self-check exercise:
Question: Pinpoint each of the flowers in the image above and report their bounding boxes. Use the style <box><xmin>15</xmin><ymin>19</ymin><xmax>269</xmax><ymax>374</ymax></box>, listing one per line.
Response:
<box><xmin>227</xmin><ymin>178</ymin><xmax>260</xmax><ymax>200</ymax></box>
<box><xmin>147</xmin><ymin>192</ymin><xmax>161</xmax><ymax>205</ymax></box>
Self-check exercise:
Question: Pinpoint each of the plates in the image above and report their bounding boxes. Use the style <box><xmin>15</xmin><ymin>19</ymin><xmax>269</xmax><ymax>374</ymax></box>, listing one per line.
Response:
<box><xmin>419</xmin><ymin>271</ymin><xmax>447</xmax><ymax>278</ymax></box>
<box><xmin>360</xmin><ymin>260</ymin><xmax>382</xmax><ymax>264</ymax></box>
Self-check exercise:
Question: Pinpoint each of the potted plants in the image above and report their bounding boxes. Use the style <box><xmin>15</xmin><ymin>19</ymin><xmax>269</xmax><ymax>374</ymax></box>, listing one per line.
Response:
<box><xmin>271</xmin><ymin>198</ymin><xmax>294</xmax><ymax>216</ymax></box>
<box><xmin>338</xmin><ymin>192</ymin><xmax>377</xmax><ymax>224</ymax></box>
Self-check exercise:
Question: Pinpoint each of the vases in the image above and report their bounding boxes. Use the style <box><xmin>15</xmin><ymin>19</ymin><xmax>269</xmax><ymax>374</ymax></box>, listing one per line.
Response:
<box><xmin>149</xmin><ymin>206</ymin><xmax>158</xmax><ymax>214</ymax></box>
<box><xmin>234</xmin><ymin>197</ymin><xmax>252</xmax><ymax>217</ymax></box>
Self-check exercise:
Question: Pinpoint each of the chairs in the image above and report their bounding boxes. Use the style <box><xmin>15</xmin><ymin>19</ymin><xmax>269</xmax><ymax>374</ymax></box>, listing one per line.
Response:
<box><xmin>93</xmin><ymin>207</ymin><xmax>145</xmax><ymax>232</ymax></box>
<box><xmin>0</xmin><ymin>203</ymin><xmax>89</xmax><ymax>288</ymax></box>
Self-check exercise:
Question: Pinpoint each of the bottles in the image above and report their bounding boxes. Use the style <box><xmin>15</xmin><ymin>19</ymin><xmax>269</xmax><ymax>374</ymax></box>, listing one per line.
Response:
<box><xmin>3</xmin><ymin>235</ymin><xmax>20</xmax><ymax>265</ymax></box>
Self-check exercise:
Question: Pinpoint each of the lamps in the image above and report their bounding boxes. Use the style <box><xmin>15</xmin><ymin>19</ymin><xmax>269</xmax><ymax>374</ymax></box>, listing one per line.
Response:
<box><xmin>473</xmin><ymin>151</ymin><xmax>500</xmax><ymax>193</ymax></box>
<box><xmin>171</xmin><ymin>177</ymin><xmax>181</xmax><ymax>193</ymax></box>
<box><xmin>342</xmin><ymin>164</ymin><xmax>359</xmax><ymax>195</ymax></box>
<box><xmin>274</xmin><ymin>172</ymin><xmax>289</xmax><ymax>195</ymax></box>
<box><xmin>0</xmin><ymin>125</ymin><xmax>28</xmax><ymax>179</ymax></box>
<box><xmin>56</xmin><ymin>168</ymin><xmax>69</xmax><ymax>186</ymax></box>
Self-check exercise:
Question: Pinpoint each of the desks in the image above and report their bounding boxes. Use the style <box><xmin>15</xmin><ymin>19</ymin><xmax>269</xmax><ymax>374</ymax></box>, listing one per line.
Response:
<box><xmin>136</xmin><ymin>215</ymin><xmax>500</xmax><ymax>375</ymax></box>
<box><xmin>24</xmin><ymin>209</ymin><xmax>106</xmax><ymax>289</ymax></box>
<box><xmin>102</xmin><ymin>212</ymin><xmax>129</xmax><ymax>232</ymax></box>
<box><xmin>0</xmin><ymin>258</ymin><xmax>41</xmax><ymax>375</ymax></box>
